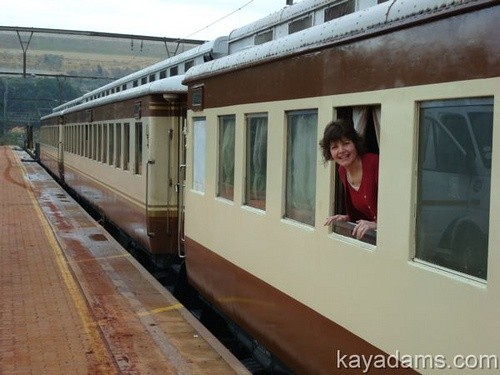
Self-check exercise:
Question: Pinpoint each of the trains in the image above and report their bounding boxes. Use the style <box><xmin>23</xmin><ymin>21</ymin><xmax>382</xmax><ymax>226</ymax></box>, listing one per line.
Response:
<box><xmin>39</xmin><ymin>0</ymin><xmax>500</xmax><ymax>375</ymax></box>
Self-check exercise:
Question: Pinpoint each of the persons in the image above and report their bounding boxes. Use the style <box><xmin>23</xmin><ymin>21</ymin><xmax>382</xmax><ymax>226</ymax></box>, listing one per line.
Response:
<box><xmin>319</xmin><ymin>118</ymin><xmax>379</xmax><ymax>240</ymax></box>
